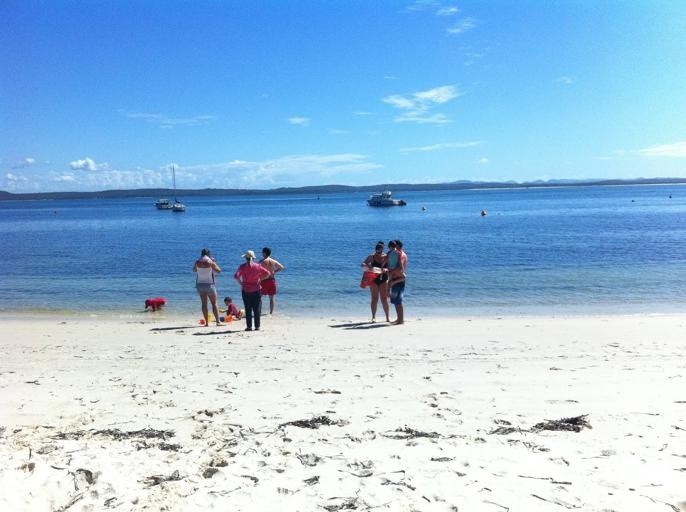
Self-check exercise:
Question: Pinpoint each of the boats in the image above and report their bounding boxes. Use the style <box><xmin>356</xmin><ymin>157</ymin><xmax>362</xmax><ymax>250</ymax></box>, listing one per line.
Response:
<box><xmin>154</xmin><ymin>162</ymin><xmax>185</xmax><ymax>213</ymax></box>
<box><xmin>365</xmin><ymin>189</ymin><xmax>401</xmax><ymax>208</ymax></box>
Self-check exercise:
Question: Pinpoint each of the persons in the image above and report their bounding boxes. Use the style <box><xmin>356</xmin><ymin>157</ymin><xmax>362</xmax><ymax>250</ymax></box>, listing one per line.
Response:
<box><xmin>234</xmin><ymin>250</ymin><xmax>271</xmax><ymax>331</ymax></box>
<box><xmin>361</xmin><ymin>241</ymin><xmax>390</xmax><ymax>322</ymax></box>
<box><xmin>380</xmin><ymin>241</ymin><xmax>406</xmax><ymax>297</ymax></box>
<box><xmin>258</xmin><ymin>247</ymin><xmax>284</xmax><ymax>315</ymax></box>
<box><xmin>193</xmin><ymin>248</ymin><xmax>225</xmax><ymax>327</ymax></box>
<box><xmin>386</xmin><ymin>240</ymin><xmax>407</xmax><ymax>325</ymax></box>
<box><xmin>145</xmin><ymin>298</ymin><xmax>166</xmax><ymax>312</ymax></box>
<box><xmin>224</xmin><ymin>297</ymin><xmax>246</xmax><ymax>318</ymax></box>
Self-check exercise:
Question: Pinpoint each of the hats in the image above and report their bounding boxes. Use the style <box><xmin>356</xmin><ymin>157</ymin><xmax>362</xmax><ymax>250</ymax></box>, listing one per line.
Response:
<box><xmin>241</xmin><ymin>250</ymin><xmax>257</xmax><ymax>260</ymax></box>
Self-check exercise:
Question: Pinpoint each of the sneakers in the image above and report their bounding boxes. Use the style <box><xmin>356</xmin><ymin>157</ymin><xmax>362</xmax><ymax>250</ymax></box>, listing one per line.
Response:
<box><xmin>245</xmin><ymin>327</ymin><xmax>260</xmax><ymax>331</ymax></box>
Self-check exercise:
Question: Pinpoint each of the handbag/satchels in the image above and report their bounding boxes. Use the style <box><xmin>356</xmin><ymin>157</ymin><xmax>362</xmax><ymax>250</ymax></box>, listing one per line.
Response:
<box><xmin>373</xmin><ymin>273</ymin><xmax>389</xmax><ymax>286</ymax></box>
